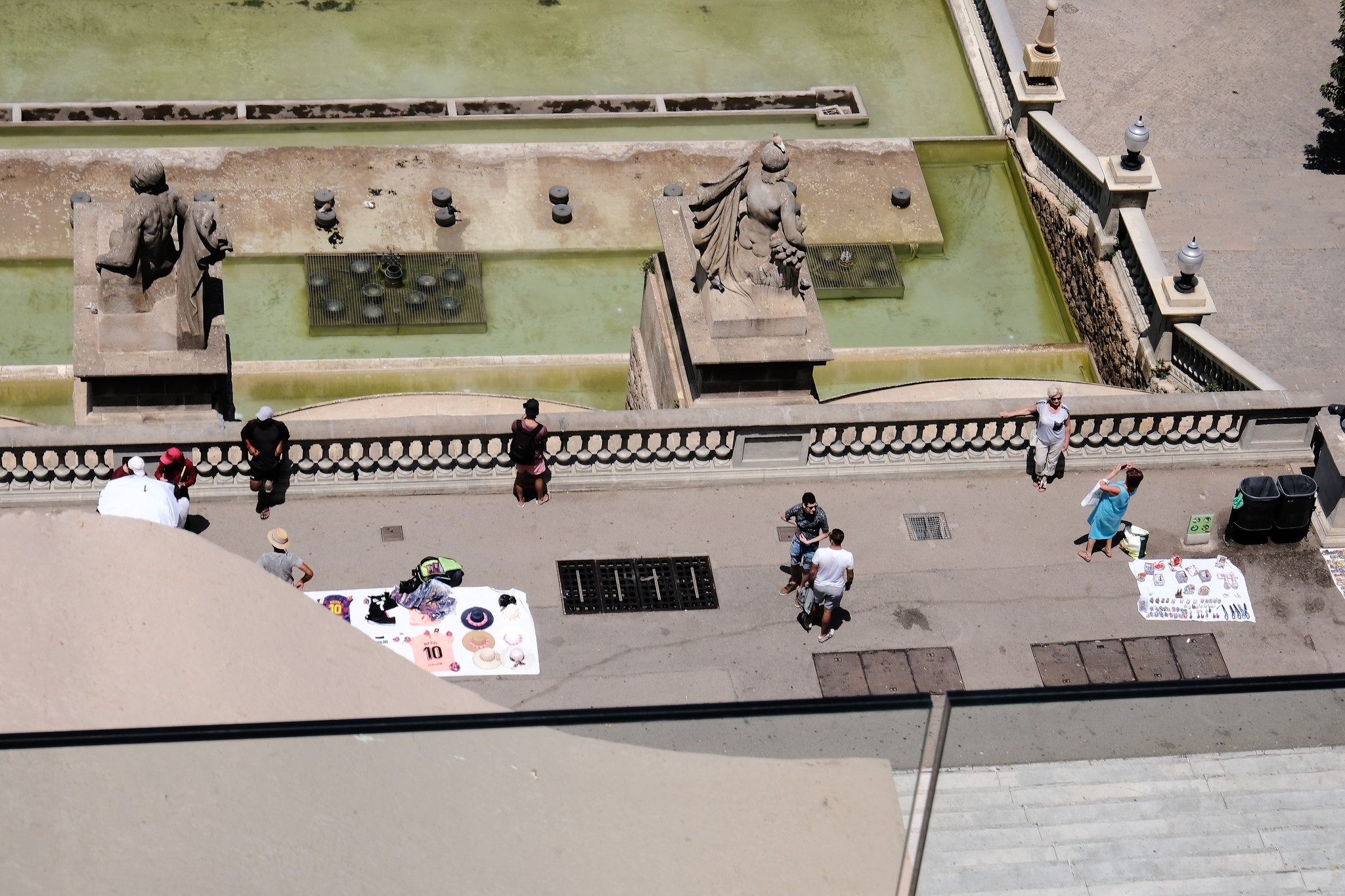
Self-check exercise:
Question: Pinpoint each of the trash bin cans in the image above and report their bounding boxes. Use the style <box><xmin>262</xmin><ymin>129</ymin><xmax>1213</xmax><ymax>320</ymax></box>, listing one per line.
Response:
<box><xmin>1269</xmin><ymin>474</ymin><xmax>1318</xmax><ymax>544</ymax></box>
<box><xmin>1224</xmin><ymin>476</ymin><xmax>1280</xmax><ymax>545</ymax></box>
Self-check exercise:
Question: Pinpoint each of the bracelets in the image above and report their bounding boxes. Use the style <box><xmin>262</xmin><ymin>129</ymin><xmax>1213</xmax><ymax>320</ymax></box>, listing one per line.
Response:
<box><xmin>160</xmin><ymin>477</ymin><xmax>162</xmax><ymax>481</ymax></box>
<box><xmin>184</xmin><ymin>482</ymin><xmax>185</xmax><ymax>487</ymax></box>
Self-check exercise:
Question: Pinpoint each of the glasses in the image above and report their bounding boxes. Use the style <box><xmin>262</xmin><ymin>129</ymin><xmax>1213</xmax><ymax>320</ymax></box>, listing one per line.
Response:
<box><xmin>807</xmin><ymin>502</ymin><xmax>818</xmax><ymax>510</ymax></box>
<box><xmin>828</xmin><ymin>533</ymin><xmax>831</xmax><ymax>539</ymax></box>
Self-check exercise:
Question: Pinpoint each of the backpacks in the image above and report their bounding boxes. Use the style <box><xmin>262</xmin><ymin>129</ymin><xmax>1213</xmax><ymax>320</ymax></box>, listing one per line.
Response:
<box><xmin>509</xmin><ymin>420</ymin><xmax>544</xmax><ymax>466</ymax></box>
<box><xmin>399</xmin><ymin>556</ymin><xmax>464</xmax><ymax>595</ymax></box>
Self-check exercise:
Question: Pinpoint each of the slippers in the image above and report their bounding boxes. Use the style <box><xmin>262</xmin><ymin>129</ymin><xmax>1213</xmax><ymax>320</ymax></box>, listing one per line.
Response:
<box><xmin>518</xmin><ymin>492</ymin><xmax>527</xmax><ymax>507</ymax></box>
<box><xmin>260</xmin><ymin>506</ymin><xmax>270</xmax><ymax>520</ymax></box>
<box><xmin>536</xmin><ymin>491</ymin><xmax>552</xmax><ymax>504</ymax></box>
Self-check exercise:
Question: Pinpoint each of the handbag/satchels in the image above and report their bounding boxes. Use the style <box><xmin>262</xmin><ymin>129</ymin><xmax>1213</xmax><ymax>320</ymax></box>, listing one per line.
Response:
<box><xmin>798</xmin><ymin>585</ymin><xmax>814</xmax><ymax>615</ymax></box>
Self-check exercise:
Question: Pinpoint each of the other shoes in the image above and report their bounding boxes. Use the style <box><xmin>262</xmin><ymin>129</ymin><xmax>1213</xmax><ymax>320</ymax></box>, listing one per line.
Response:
<box><xmin>818</xmin><ymin>628</ymin><xmax>835</xmax><ymax>643</ymax></box>
<box><xmin>794</xmin><ymin>590</ymin><xmax>800</xmax><ymax>608</ymax></box>
<box><xmin>779</xmin><ymin>582</ymin><xmax>800</xmax><ymax>596</ymax></box>
<box><xmin>801</xmin><ymin>611</ymin><xmax>812</xmax><ymax>630</ymax></box>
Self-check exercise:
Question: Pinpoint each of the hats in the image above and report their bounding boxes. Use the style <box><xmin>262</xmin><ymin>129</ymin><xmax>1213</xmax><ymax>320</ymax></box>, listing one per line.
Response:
<box><xmin>495</xmin><ymin>627</ymin><xmax>525</xmax><ymax>649</ymax></box>
<box><xmin>255</xmin><ymin>405</ymin><xmax>273</xmax><ymax>421</ymax></box>
<box><xmin>502</xmin><ymin>603</ymin><xmax>522</xmax><ymax>621</ymax></box>
<box><xmin>267</xmin><ymin>527</ymin><xmax>290</xmax><ymax>549</ymax></box>
<box><xmin>499</xmin><ymin>594</ymin><xmax>516</xmax><ymax>607</ymax></box>
<box><xmin>505</xmin><ymin>645</ymin><xmax>534</xmax><ymax>670</ymax></box>
<box><xmin>128</xmin><ymin>455</ymin><xmax>145</xmax><ymax>476</ymax></box>
<box><xmin>472</xmin><ymin>647</ymin><xmax>501</xmax><ymax>670</ymax></box>
<box><xmin>461</xmin><ymin>606</ymin><xmax>494</xmax><ymax>629</ymax></box>
<box><xmin>523</xmin><ymin>398</ymin><xmax>539</xmax><ymax>416</ymax></box>
<box><xmin>462</xmin><ymin>630</ymin><xmax>495</xmax><ymax>653</ymax></box>
<box><xmin>160</xmin><ymin>447</ymin><xmax>183</xmax><ymax>465</ymax></box>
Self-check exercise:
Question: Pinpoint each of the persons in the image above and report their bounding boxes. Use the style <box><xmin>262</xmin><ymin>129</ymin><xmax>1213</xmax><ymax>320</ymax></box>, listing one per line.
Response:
<box><xmin>511</xmin><ymin>399</ymin><xmax>550</xmax><ymax>508</ymax></box>
<box><xmin>154</xmin><ymin>448</ymin><xmax>197</xmax><ymax>528</ymax></box>
<box><xmin>256</xmin><ymin>527</ymin><xmax>314</xmax><ymax>592</ymax></box>
<box><xmin>241</xmin><ymin>406</ymin><xmax>290</xmax><ymax>519</ymax></box>
<box><xmin>778</xmin><ymin>492</ymin><xmax>830</xmax><ymax>607</ymax></box>
<box><xmin>110</xmin><ymin>455</ymin><xmax>147</xmax><ymax>480</ymax></box>
<box><xmin>736</xmin><ymin>143</ymin><xmax>806</xmax><ymax>256</ymax></box>
<box><xmin>1077</xmin><ymin>463</ymin><xmax>1144</xmax><ymax>562</ymax></box>
<box><xmin>94</xmin><ymin>156</ymin><xmax>189</xmax><ymax>287</ymax></box>
<box><xmin>1000</xmin><ymin>384</ymin><xmax>1071</xmax><ymax>493</ymax></box>
<box><xmin>800</xmin><ymin>528</ymin><xmax>854</xmax><ymax>643</ymax></box>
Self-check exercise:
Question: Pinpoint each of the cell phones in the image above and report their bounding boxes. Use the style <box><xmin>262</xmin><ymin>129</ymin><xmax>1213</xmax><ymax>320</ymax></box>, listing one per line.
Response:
<box><xmin>1120</xmin><ymin>464</ymin><xmax>1128</xmax><ymax>470</ymax></box>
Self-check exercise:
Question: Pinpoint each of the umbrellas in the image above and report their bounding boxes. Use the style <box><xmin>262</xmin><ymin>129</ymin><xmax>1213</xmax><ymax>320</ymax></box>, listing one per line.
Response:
<box><xmin>96</xmin><ymin>474</ymin><xmax>181</xmax><ymax>528</ymax></box>
